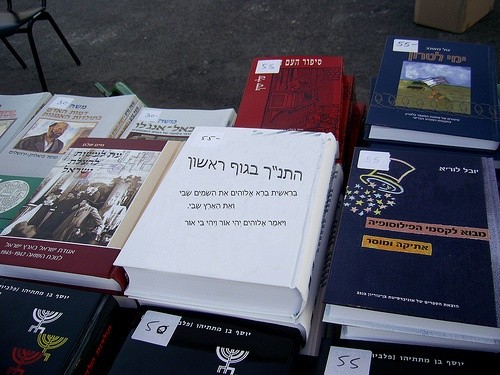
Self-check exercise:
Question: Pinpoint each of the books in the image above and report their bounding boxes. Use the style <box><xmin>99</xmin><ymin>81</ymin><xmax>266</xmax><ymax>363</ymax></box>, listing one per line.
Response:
<box><xmin>365</xmin><ymin>34</ymin><xmax>500</xmax><ymax>151</ymax></box>
<box><xmin>0</xmin><ymin>33</ymin><xmax>500</xmax><ymax>375</ymax></box>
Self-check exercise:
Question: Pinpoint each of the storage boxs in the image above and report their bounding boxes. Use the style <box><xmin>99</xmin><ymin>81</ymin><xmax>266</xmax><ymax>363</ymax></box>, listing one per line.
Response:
<box><xmin>413</xmin><ymin>0</ymin><xmax>494</xmax><ymax>32</ymax></box>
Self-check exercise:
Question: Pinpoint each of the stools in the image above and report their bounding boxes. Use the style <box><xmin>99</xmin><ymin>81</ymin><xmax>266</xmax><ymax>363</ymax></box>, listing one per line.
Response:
<box><xmin>0</xmin><ymin>0</ymin><xmax>83</xmax><ymax>93</ymax></box>
